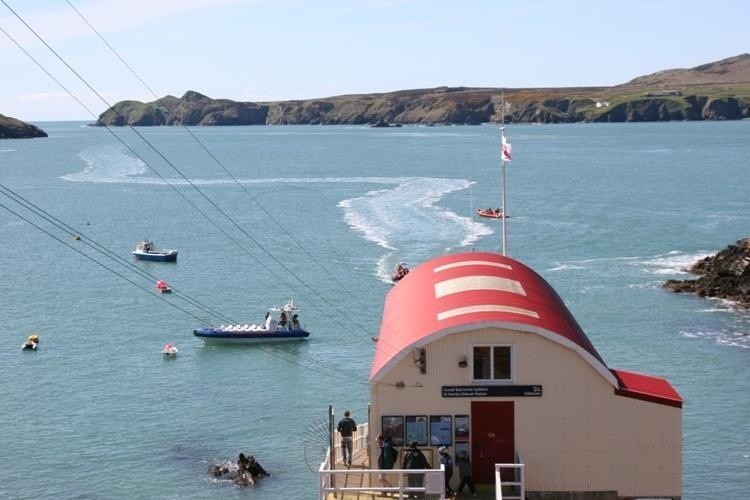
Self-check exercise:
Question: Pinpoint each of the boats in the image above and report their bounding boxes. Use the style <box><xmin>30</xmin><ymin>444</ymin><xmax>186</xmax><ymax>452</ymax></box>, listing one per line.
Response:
<box><xmin>161</xmin><ymin>343</ymin><xmax>178</xmax><ymax>358</ymax></box>
<box><xmin>191</xmin><ymin>297</ymin><xmax>315</xmax><ymax>345</ymax></box>
<box><xmin>131</xmin><ymin>236</ymin><xmax>181</xmax><ymax>264</ymax></box>
<box><xmin>390</xmin><ymin>262</ymin><xmax>410</xmax><ymax>283</ymax></box>
<box><xmin>476</xmin><ymin>204</ymin><xmax>511</xmax><ymax>221</ymax></box>
<box><xmin>20</xmin><ymin>339</ymin><xmax>37</xmax><ymax>350</ymax></box>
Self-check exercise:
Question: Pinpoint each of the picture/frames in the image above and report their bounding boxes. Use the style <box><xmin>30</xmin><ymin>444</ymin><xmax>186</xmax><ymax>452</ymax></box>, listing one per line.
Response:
<box><xmin>380</xmin><ymin>413</ymin><xmax>472</xmax><ymax>471</ymax></box>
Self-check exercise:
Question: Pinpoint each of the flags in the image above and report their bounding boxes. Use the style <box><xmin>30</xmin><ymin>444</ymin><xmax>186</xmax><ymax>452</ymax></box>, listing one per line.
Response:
<box><xmin>500</xmin><ymin>133</ymin><xmax>511</xmax><ymax>162</ymax></box>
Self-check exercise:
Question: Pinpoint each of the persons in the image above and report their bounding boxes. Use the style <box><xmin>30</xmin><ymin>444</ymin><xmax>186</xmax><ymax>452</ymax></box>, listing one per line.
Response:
<box><xmin>403</xmin><ymin>442</ymin><xmax>433</xmax><ymax>499</ymax></box>
<box><xmin>455</xmin><ymin>451</ymin><xmax>477</xmax><ymax>498</ymax></box>
<box><xmin>376</xmin><ymin>434</ymin><xmax>396</xmax><ymax>497</ymax></box>
<box><xmin>336</xmin><ymin>410</ymin><xmax>357</xmax><ymax>468</ymax></box>
<box><xmin>278</xmin><ymin>313</ymin><xmax>286</xmax><ymax>326</ymax></box>
<box><xmin>289</xmin><ymin>314</ymin><xmax>300</xmax><ymax>329</ymax></box>
<box><xmin>398</xmin><ymin>266</ymin><xmax>402</xmax><ymax>274</ymax></box>
<box><xmin>439</xmin><ymin>446</ymin><xmax>454</xmax><ymax>497</ymax></box>
